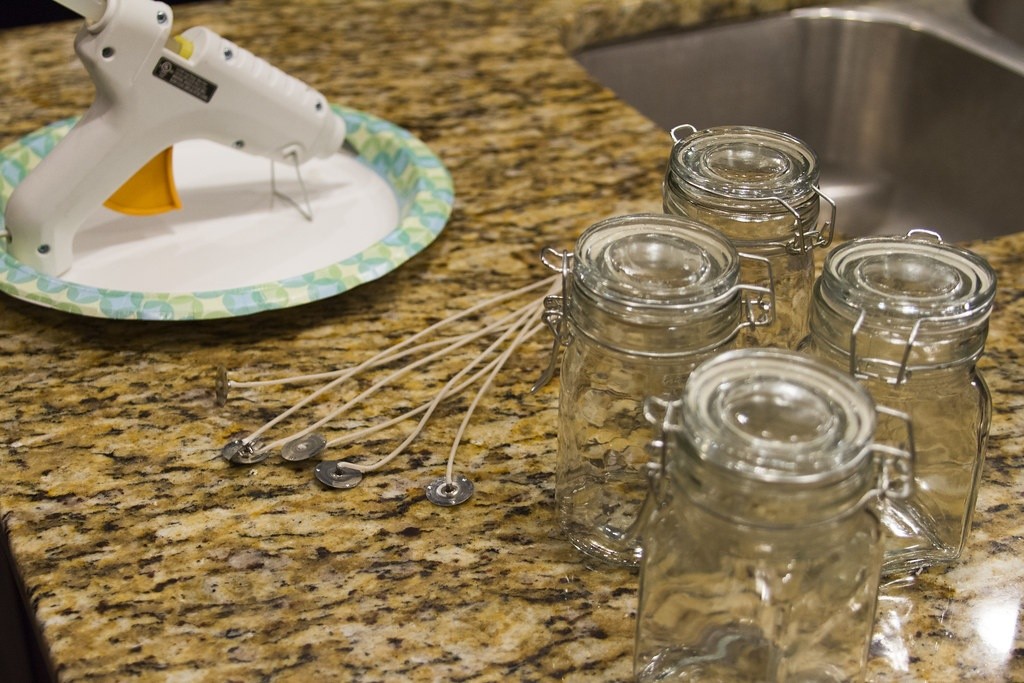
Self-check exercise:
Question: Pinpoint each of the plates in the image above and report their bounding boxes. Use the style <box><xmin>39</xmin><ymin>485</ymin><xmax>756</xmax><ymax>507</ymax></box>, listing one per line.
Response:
<box><xmin>0</xmin><ymin>103</ymin><xmax>454</xmax><ymax>320</ymax></box>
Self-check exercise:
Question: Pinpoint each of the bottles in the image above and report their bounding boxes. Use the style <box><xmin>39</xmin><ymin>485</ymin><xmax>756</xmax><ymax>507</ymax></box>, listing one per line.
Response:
<box><xmin>541</xmin><ymin>119</ymin><xmax>998</xmax><ymax>683</ymax></box>
